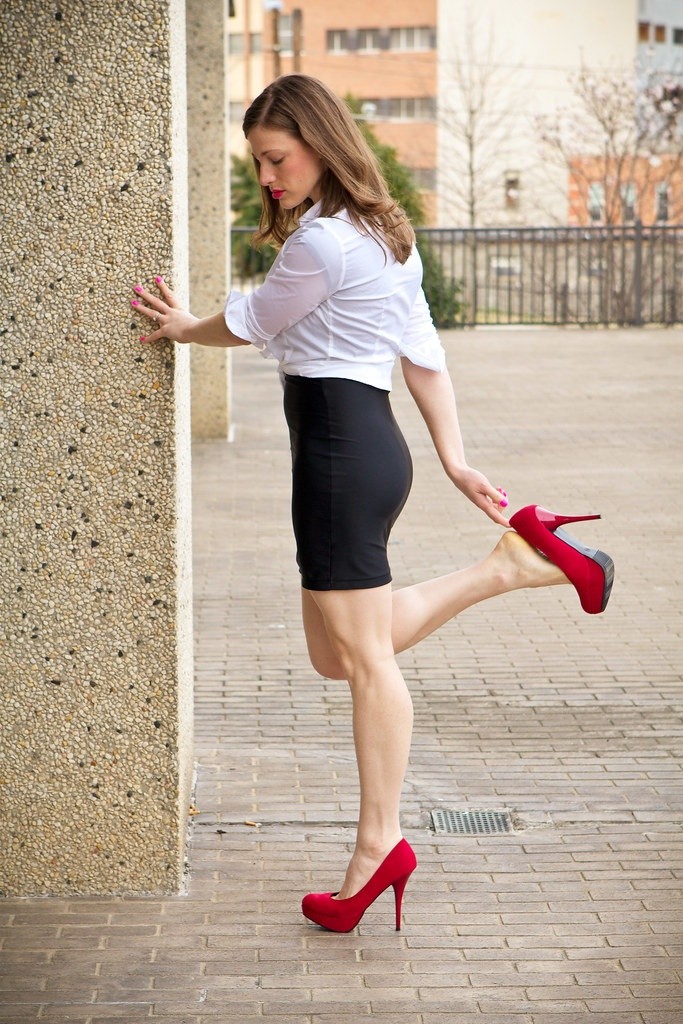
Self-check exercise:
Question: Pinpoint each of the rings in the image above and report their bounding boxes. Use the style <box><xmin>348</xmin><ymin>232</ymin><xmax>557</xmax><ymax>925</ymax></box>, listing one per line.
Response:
<box><xmin>153</xmin><ymin>313</ymin><xmax>162</xmax><ymax>321</ymax></box>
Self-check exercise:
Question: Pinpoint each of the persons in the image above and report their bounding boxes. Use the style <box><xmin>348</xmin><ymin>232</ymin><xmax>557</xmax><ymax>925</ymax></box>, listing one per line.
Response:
<box><xmin>129</xmin><ymin>76</ymin><xmax>615</xmax><ymax>933</ymax></box>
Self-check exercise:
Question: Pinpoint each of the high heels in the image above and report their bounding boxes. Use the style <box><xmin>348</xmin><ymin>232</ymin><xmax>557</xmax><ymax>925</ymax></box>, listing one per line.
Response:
<box><xmin>508</xmin><ymin>504</ymin><xmax>614</xmax><ymax>614</ymax></box>
<box><xmin>302</xmin><ymin>838</ymin><xmax>417</xmax><ymax>932</ymax></box>
